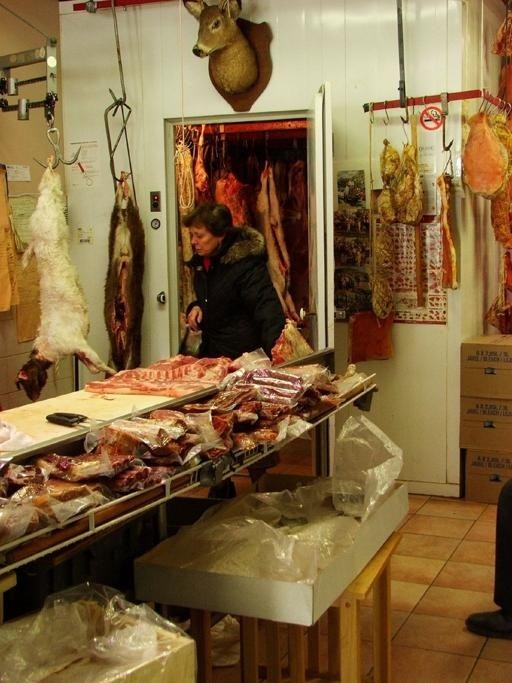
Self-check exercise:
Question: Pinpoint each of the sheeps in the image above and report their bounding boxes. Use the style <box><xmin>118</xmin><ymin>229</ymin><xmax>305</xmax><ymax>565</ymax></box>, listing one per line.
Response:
<box><xmin>16</xmin><ymin>156</ymin><xmax>117</xmax><ymax>402</ymax></box>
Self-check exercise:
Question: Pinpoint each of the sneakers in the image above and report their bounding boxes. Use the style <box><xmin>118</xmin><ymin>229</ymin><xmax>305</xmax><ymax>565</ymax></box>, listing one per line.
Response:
<box><xmin>464</xmin><ymin>608</ymin><xmax>512</xmax><ymax>641</ymax></box>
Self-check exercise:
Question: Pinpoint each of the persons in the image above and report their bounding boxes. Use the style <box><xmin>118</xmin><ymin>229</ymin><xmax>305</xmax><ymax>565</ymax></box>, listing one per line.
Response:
<box><xmin>463</xmin><ymin>478</ymin><xmax>512</xmax><ymax>640</ymax></box>
<box><xmin>185</xmin><ymin>202</ymin><xmax>283</xmax><ymax>498</ymax></box>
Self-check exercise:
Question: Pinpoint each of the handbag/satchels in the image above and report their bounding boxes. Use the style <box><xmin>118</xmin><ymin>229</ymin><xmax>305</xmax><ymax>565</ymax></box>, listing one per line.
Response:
<box><xmin>175</xmin><ymin>323</ymin><xmax>203</xmax><ymax>358</ymax></box>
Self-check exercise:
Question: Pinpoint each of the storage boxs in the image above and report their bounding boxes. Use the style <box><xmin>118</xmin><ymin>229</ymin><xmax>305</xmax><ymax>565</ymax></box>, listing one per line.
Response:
<box><xmin>129</xmin><ymin>475</ymin><xmax>416</xmax><ymax>628</ymax></box>
<box><xmin>465</xmin><ymin>450</ymin><xmax>512</xmax><ymax>503</ymax></box>
<box><xmin>460</xmin><ymin>332</ymin><xmax>512</xmax><ymax>399</ymax></box>
<box><xmin>460</xmin><ymin>396</ymin><xmax>512</xmax><ymax>454</ymax></box>
<box><xmin>0</xmin><ymin>597</ymin><xmax>196</xmax><ymax>682</ymax></box>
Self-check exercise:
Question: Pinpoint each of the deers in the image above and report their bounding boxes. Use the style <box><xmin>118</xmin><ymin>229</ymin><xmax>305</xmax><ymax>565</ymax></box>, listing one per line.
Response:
<box><xmin>181</xmin><ymin>0</ymin><xmax>260</xmax><ymax>98</ymax></box>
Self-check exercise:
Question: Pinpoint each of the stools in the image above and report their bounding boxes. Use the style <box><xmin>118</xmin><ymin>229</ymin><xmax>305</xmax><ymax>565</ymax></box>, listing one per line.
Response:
<box><xmin>201</xmin><ymin>531</ymin><xmax>404</xmax><ymax>682</ymax></box>
